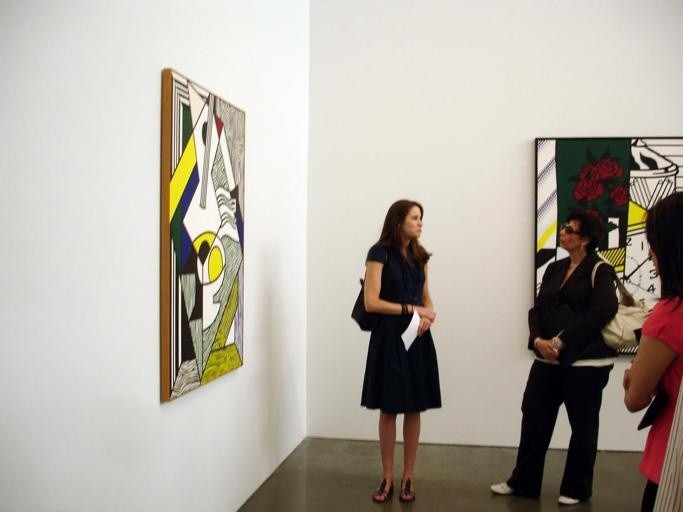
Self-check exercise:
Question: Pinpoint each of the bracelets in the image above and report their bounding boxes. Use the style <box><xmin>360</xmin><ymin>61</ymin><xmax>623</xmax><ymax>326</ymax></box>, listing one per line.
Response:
<box><xmin>400</xmin><ymin>302</ymin><xmax>415</xmax><ymax>317</ymax></box>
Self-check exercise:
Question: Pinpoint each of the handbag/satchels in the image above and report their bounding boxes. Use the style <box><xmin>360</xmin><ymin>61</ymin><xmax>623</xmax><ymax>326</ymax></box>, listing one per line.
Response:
<box><xmin>351</xmin><ymin>279</ymin><xmax>377</xmax><ymax>331</ymax></box>
<box><xmin>601</xmin><ymin>298</ymin><xmax>649</xmax><ymax>347</ymax></box>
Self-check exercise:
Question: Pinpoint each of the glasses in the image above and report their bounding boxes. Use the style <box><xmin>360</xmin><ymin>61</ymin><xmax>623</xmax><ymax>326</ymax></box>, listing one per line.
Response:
<box><xmin>559</xmin><ymin>223</ymin><xmax>577</xmax><ymax>235</ymax></box>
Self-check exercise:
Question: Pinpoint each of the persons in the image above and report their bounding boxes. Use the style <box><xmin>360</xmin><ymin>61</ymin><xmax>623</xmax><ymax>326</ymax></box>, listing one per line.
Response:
<box><xmin>359</xmin><ymin>200</ymin><xmax>444</xmax><ymax>504</ymax></box>
<box><xmin>488</xmin><ymin>205</ymin><xmax>620</xmax><ymax>506</ymax></box>
<box><xmin>621</xmin><ymin>190</ymin><xmax>683</xmax><ymax>512</ymax></box>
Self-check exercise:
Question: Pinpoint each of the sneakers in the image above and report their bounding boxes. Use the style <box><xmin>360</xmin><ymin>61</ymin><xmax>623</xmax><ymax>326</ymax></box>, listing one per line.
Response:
<box><xmin>558</xmin><ymin>496</ymin><xmax>580</xmax><ymax>505</ymax></box>
<box><xmin>491</xmin><ymin>482</ymin><xmax>515</xmax><ymax>495</ymax></box>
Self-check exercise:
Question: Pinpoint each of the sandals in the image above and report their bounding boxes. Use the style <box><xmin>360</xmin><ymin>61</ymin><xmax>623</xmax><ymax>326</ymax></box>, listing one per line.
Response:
<box><xmin>372</xmin><ymin>478</ymin><xmax>393</xmax><ymax>503</ymax></box>
<box><xmin>400</xmin><ymin>479</ymin><xmax>415</xmax><ymax>503</ymax></box>
